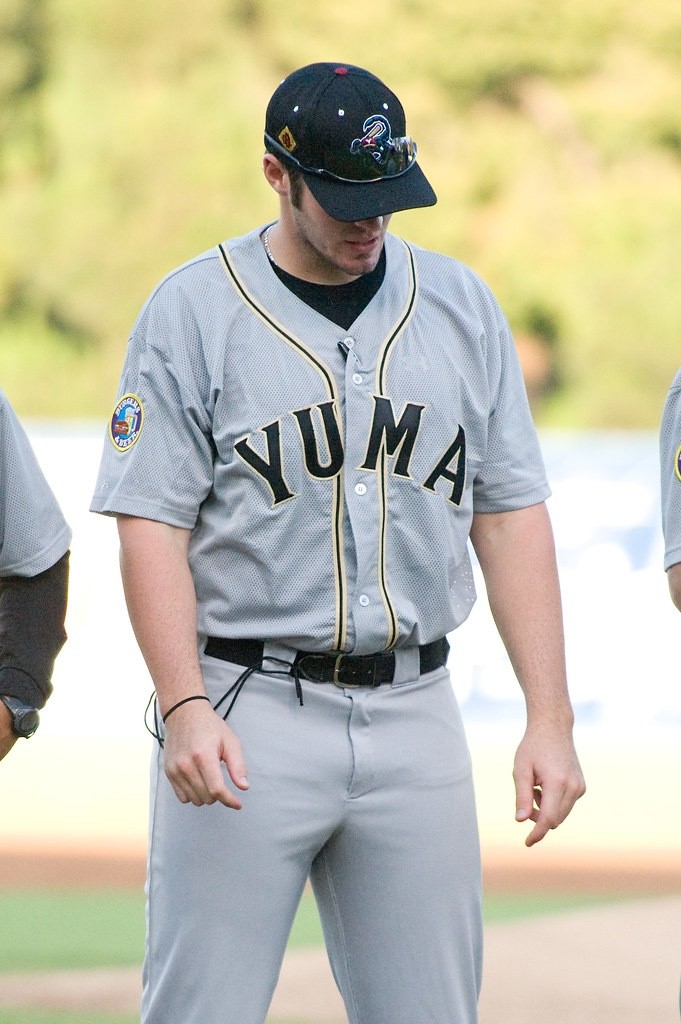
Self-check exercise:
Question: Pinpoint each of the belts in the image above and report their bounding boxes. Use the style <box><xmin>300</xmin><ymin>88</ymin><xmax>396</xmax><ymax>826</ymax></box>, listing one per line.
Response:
<box><xmin>205</xmin><ymin>636</ymin><xmax>450</xmax><ymax>689</ymax></box>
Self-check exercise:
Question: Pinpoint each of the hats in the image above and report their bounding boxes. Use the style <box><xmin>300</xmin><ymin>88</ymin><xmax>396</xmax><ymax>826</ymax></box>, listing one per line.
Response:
<box><xmin>264</xmin><ymin>62</ymin><xmax>437</xmax><ymax>223</ymax></box>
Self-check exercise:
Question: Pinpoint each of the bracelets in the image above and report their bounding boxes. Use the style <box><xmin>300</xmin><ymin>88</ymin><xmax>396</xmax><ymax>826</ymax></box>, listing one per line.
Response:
<box><xmin>163</xmin><ymin>696</ymin><xmax>210</xmax><ymax>724</ymax></box>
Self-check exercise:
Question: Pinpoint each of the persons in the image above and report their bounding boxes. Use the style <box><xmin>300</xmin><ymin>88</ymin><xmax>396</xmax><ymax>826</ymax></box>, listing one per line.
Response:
<box><xmin>659</xmin><ymin>369</ymin><xmax>681</xmax><ymax>613</ymax></box>
<box><xmin>89</xmin><ymin>62</ymin><xmax>587</xmax><ymax>1023</ymax></box>
<box><xmin>0</xmin><ymin>387</ymin><xmax>73</xmax><ymax>761</ymax></box>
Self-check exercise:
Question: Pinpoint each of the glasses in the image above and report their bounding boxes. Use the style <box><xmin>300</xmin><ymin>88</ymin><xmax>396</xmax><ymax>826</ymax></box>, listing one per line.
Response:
<box><xmin>264</xmin><ymin>127</ymin><xmax>418</xmax><ymax>181</ymax></box>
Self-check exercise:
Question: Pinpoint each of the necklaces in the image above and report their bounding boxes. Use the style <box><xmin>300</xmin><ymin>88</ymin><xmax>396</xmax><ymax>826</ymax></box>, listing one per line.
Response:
<box><xmin>265</xmin><ymin>224</ymin><xmax>277</xmax><ymax>265</ymax></box>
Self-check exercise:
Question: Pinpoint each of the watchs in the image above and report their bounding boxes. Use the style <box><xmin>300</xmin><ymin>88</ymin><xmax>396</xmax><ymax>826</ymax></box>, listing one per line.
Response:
<box><xmin>0</xmin><ymin>695</ymin><xmax>40</xmax><ymax>738</ymax></box>
